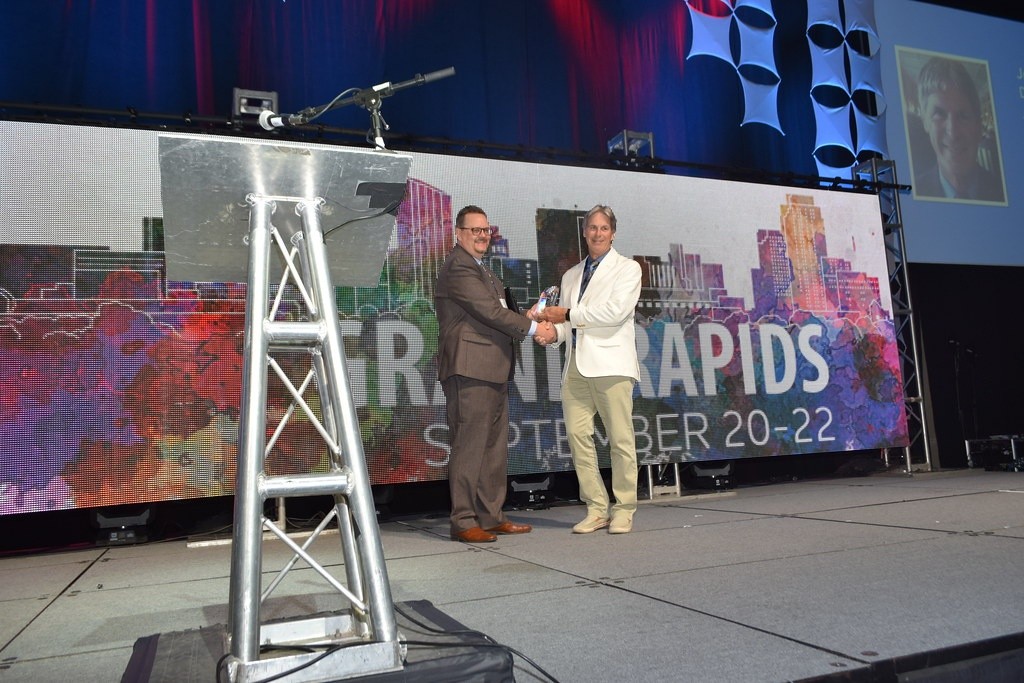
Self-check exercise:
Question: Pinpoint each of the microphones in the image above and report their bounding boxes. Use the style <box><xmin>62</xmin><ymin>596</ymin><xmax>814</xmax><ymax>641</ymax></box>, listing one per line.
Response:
<box><xmin>259</xmin><ymin>110</ymin><xmax>308</xmax><ymax>130</ymax></box>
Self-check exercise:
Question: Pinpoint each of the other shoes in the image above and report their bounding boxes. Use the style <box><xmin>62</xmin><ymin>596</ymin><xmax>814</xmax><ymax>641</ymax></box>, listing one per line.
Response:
<box><xmin>609</xmin><ymin>514</ymin><xmax>632</xmax><ymax>533</ymax></box>
<box><xmin>573</xmin><ymin>515</ymin><xmax>612</xmax><ymax>533</ymax></box>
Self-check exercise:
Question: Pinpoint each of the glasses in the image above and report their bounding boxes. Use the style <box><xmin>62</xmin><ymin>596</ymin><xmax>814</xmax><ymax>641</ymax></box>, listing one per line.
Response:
<box><xmin>460</xmin><ymin>227</ymin><xmax>493</xmax><ymax>236</ymax></box>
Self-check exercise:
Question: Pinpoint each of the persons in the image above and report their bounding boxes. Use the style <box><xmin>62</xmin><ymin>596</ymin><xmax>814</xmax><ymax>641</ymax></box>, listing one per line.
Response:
<box><xmin>915</xmin><ymin>56</ymin><xmax>1005</xmax><ymax>202</ymax></box>
<box><xmin>532</xmin><ymin>204</ymin><xmax>642</xmax><ymax>534</ymax></box>
<box><xmin>434</xmin><ymin>205</ymin><xmax>556</xmax><ymax>542</ymax></box>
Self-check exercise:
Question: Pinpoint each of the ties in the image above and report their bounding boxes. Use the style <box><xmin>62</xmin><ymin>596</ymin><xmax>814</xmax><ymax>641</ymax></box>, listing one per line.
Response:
<box><xmin>480</xmin><ymin>263</ymin><xmax>502</xmax><ymax>299</ymax></box>
<box><xmin>578</xmin><ymin>261</ymin><xmax>599</xmax><ymax>304</ymax></box>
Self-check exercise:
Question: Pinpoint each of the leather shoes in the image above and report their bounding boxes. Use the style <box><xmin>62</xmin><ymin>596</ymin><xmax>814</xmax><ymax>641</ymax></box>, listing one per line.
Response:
<box><xmin>451</xmin><ymin>527</ymin><xmax>497</xmax><ymax>543</ymax></box>
<box><xmin>483</xmin><ymin>520</ymin><xmax>532</xmax><ymax>535</ymax></box>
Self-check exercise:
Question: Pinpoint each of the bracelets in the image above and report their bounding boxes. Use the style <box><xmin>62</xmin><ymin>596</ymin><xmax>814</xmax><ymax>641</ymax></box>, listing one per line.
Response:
<box><xmin>565</xmin><ymin>309</ymin><xmax>570</xmax><ymax>321</ymax></box>
